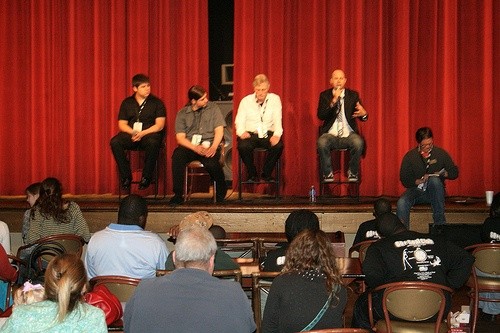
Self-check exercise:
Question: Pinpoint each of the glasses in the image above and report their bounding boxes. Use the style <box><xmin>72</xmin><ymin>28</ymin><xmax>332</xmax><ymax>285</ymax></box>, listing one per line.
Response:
<box><xmin>419</xmin><ymin>140</ymin><xmax>433</xmax><ymax>147</ymax></box>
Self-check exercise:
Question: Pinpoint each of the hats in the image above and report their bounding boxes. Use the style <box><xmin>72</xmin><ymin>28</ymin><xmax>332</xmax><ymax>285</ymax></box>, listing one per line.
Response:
<box><xmin>179</xmin><ymin>211</ymin><xmax>212</xmax><ymax>232</ymax></box>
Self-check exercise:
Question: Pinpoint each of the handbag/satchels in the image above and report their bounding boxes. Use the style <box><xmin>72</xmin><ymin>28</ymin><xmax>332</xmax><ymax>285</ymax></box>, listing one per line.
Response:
<box><xmin>84</xmin><ymin>286</ymin><xmax>123</xmax><ymax>327</ymax></box>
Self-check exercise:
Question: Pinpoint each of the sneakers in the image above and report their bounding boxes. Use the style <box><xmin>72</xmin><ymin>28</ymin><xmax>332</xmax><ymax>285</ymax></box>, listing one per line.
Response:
<box><xmin>323</xmin><ymin>171</ymin><xmax>335</xmax><ymax>183</ymax></box>
<box><xmin>347</xmin><ymin>169</ymin><xmax>359</xmax><ymax>181</ymax></box>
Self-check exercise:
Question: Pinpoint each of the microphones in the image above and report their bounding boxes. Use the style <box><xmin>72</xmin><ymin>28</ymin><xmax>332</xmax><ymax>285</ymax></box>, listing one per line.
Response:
<box><xmin>337</xmin><ymin>85</ymin><xmax>341</xmax><ymax>99</ymax></box>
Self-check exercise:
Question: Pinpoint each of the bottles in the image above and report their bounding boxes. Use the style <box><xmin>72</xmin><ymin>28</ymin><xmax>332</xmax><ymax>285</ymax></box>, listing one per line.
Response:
<box><xmin>309</xmin><ymin>185</ymin><xmax>316</xmax><ymax>202</ymax></box>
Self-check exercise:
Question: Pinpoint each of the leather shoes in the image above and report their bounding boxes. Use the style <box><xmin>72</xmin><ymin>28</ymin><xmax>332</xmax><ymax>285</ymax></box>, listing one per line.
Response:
<box><xmin>139</xmin><ymin>177</ymin><xmax>150</xmax><ymax>190</ymax></box>
<box><xmin>122</xmin><ymin>176</ymin><xmax>130</xmax><ymax>189</ymax></box>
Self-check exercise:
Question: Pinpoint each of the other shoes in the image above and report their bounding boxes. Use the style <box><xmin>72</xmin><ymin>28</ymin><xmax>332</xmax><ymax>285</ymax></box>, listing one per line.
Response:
<box><xmin>261</xmin><ymin>172</ymin><xmax>275</xmax><ymax>183</ymax></box>
<box><xmin>170</xmin><ymin>195</ymin><xmax>185</xmax><ymax>205</ymax></box>
<box><xmin>211</xmin><ymin>196</ymin><xmax>224</xmax><ymax>205</ymax></box>
<box><xmin>247</xmin><ymin>169</ymin><xmax>257</xmax><ymax>182</ymax></box>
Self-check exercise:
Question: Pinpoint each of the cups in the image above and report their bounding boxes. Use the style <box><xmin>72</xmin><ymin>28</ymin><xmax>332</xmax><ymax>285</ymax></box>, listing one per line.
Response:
<box><xmin>485</xmin><ymin>190</ymin><xmax>494</xmax><ymax>203</ymax></box>
<box><xmin>202</xmin><ymin>141</ymin><xmax>211</xmax><ymax>149</ymax></box>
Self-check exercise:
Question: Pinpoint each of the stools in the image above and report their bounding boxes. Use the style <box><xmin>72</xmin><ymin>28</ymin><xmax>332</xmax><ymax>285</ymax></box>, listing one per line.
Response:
<box><xmin>318</xmin><ymin>125</ymin><xmax>360</xmax><ymax>201</ymax></box>
<box><xmin>116</xmin><ymin>117</ymin><xmax>166</xmax><ymax>201</ymax></box>
<box><xmin>237</xmin><ymin>134</ymin><xmax>280</xmax><ymax>201</ymax></box>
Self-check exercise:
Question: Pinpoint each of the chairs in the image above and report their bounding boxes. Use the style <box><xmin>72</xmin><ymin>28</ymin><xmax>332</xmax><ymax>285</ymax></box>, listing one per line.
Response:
<box><xmin>0</xmin><ymin>237</ymin><xmax>500</xmax><ymax>333</ymax></box>
<box><xmin>183</xmin><ymin>137</ymin><xmax>225</xmax><ymax>203</ymax></box>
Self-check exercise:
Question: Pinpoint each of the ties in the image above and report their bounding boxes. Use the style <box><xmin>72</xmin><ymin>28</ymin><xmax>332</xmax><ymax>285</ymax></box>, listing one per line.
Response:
<box><xmin>336</xmin><ymin>96</ymin><xmax>344</xmax><ymax>138</ymax></box>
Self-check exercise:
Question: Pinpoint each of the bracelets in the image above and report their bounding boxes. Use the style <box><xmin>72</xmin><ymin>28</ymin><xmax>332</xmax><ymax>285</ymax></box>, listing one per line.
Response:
<box><xmin>362</xmin><ymin>114</ymin><xmax>367</xmax><ymax>118</ymax></box>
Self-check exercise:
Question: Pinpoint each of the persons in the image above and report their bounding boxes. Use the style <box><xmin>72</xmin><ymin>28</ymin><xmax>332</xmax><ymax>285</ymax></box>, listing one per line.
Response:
<box><xmin>353</xmin><ymin>212</ymin><xmax>475</xmax><ymax>333</ymax></box>
<box><xmin>317</xmin><ymin>70</ymin><xmax>368</xmax><ymax>182</ymax></box>
<box><xmin>165</xmin><ymin>225</ymin><xmax>242</xmax><ymax>279</ymax></box>
<box><xmin>170</xmin><ymin>87</ymin><xmax>227</xmax><ymax>204</ymax></box>
<box><xmin>264</xmin><ymin>209</ymin><xmax>319</xmax><ymax>281</ymax></box>
<box><xmin>124</xmin><ymin>227</ymin><xmax>257</xmax><ymax>333</ymax></box>
<box><xmin>263</xmin><ymin>228</ymin><xmax>348</xmax><ymax>333</ymax></box>
<box><xmin>85</xmin><ymin>195</ymin><xmax>170</xmax><ymax>281</ymax></box>
<box><xmin>28</xmin><ymin>177</ymin><xmax>90</xmax><ymax>267</ymax></box>
<box><xmin>0</xmin><ymin>221</ymin><xmax>17</xmax><ymax>312</ymax></box>
<box><xmin>353</xmin><ymin>199</ymin><xmax>392</xmax><ymax>251</ymax></box>
<box><xmin>0</xmin><ymin>253</ymin><xmax>108</xmax><ymax>333</ymax></box>
<box><xmin>12</xmin><ymin>281</ymin><xmax>46</xmax><ymax>309</ymax></box>
<box><xmin>23</xmin><ymin>183</ymin><xmax>40</xmax><ymax>244</ymax></box>
<box><xmin>481</xmin><ymin>192</ymin><xmax>500</xmax><ymax>243</ymax></box>
<box><xmin>235</xmin><ymin>74</ymin><xmax>284</xmax><ymax>182</ymax></box>
<box><xmin>110</xmin><ymin>75</ymin><xmax>167</xmax><ymax>190</ymax></box>
<box><xmin>397</xmin><ymin>127</ymin><xmax>458</xmax><ymax>229</ymax></box>
